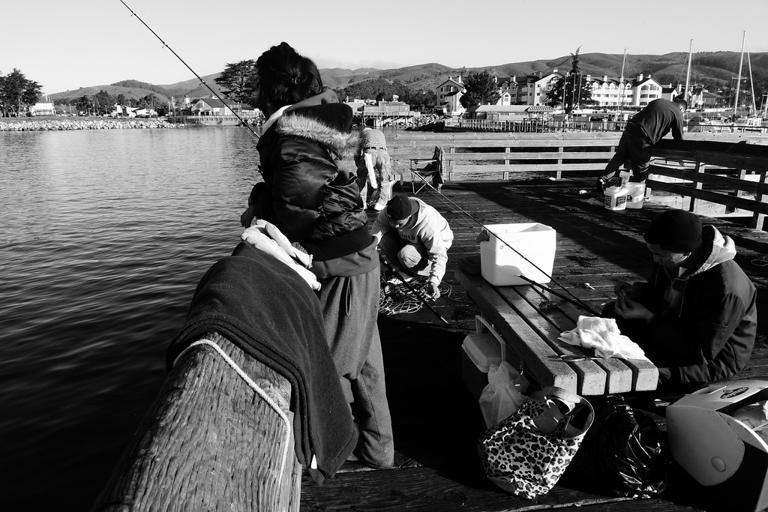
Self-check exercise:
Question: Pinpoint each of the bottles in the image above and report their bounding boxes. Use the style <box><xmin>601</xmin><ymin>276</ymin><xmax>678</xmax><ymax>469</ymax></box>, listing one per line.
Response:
<box><xmin>387</xmin><ymin>195</ymin><xmax>419</xmax><ymax>220</ymax></box>
<box><xmin>643</xmin><ymin>210</ymin><xmax>701</xmax><ymax>250</ymax></box>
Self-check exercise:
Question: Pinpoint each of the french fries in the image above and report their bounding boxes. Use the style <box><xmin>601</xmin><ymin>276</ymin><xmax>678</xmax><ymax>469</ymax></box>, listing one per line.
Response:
<box><xmin>120</xmin><ymin>0</ymin><xmax>448</xmax><ymax>325</ymax></box>
<box><xmin>513</xmin><ymin>271</ymin><xmax>602</xmax><ymax>317</ymax></box>
<box><xmin>386</xmin><ymin>147</ymin><xmax>601</xmax><ymax>317</ymax></box>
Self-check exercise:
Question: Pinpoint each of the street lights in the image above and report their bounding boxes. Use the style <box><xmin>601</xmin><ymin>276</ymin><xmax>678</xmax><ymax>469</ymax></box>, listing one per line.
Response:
<box><xmin>478</xmin><ymin>385</ymin><xmax>595</xmax><ymax>501</ymax></box>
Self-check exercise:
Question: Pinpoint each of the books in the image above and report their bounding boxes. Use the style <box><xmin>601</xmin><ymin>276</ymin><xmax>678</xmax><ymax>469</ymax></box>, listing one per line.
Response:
<box><xmin>461</xmin><ymin>313</ymin><xmax>515</xmax><ymax>400</ymax></box>
<box><xmin>482</xmin><ymin>223</ymin><xmax>556</xmax><ymax>286</ymax></box>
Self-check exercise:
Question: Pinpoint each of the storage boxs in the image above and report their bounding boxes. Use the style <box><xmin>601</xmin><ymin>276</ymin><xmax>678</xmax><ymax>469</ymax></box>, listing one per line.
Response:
<box><xmin>454</xmin><ymin>255</ymin><xmax>659</xmax><ymax>395</ymax></box>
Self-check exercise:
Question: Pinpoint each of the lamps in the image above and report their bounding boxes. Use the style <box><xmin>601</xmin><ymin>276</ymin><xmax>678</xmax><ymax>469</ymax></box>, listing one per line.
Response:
<box><xmin>409</xmin><ymin>145</ymin><xmax>445</xmax><ymax>195</ymax></box>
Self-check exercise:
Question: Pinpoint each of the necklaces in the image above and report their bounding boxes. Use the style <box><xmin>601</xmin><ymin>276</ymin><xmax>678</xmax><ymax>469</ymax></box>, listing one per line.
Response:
<box><xmin>681</xmin><ymin>31</ymin><xmax>766</xmax><ymax>134</ymax></box>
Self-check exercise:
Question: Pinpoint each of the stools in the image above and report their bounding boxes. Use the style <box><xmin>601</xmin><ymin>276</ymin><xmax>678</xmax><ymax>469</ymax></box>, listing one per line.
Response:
<box><xmin>364</xmin><ymin>146</ymin><xmax>386</xmax><ymax>149</ymax></box>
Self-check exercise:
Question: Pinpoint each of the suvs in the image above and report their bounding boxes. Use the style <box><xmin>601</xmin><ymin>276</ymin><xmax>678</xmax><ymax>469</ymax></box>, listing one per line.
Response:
<box><xmin>603</xmin><ymin>185</ymin><xmax>627</xmax><ymax>211</ymax></box>
<box><xmin>625</xmin><ymin>181</ymin><xmax>647</xmax><ymax>210</ymax></box>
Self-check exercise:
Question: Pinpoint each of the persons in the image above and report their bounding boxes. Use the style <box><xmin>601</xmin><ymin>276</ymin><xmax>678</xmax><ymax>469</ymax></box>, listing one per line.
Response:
<box><xmin>600</xmin><ymin>208</ymin><xmax>760</xmax><ymax>418</ymax></box>
<box><xmin>371</xmin><ymin>193</ymin><xmax>455</xmax><ymax>300</ymax></box>
<box><xmin>353</xmin><ymin>124</ymin><xmax>392</xmax><ymax>214</ymax></box>
<box><xmin>597</xmin><ymin>97</ymin><xmax>688</xmax><ymax>205</ymax></box>
<box><xmin>238</xmin><ymin>40</ymin><xmax>397</xmax><ymax>471</ymax></box>
